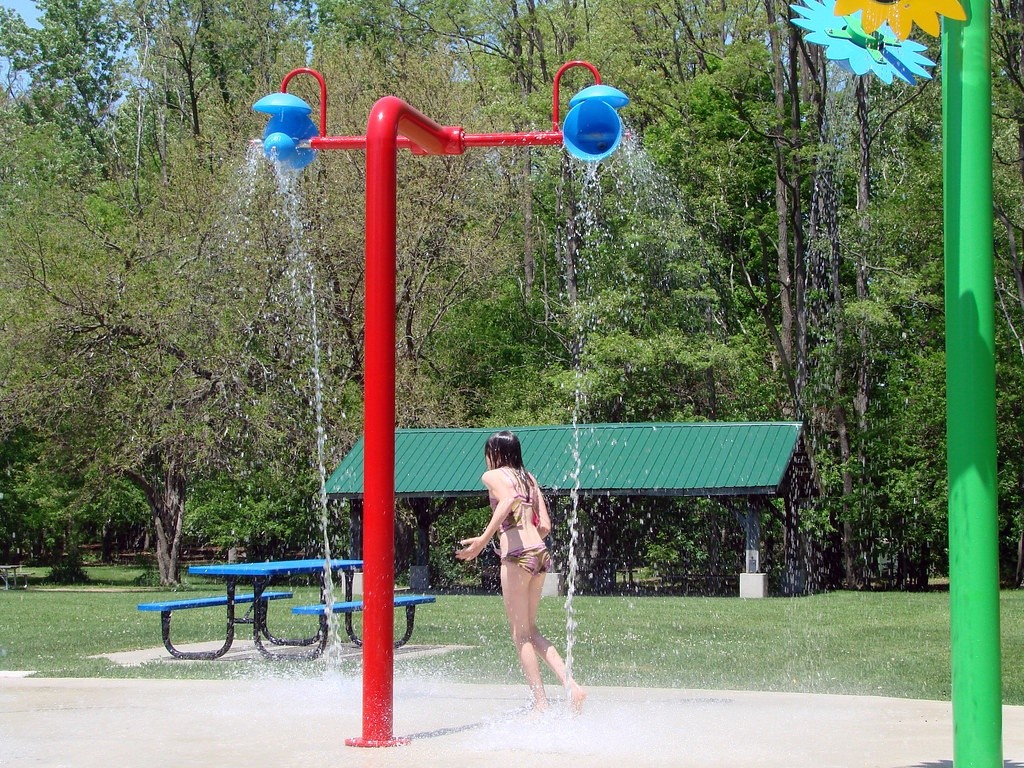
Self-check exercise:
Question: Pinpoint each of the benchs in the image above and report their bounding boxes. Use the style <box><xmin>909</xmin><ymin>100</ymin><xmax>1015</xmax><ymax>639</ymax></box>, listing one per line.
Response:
<box><xmin>292</xmin><ymin>596</ymin><xmax>436</xmax><ymax>614</ymax></box>
<box><xmin>139</xmin><ymin>592</ymin><xmax>293</xmax><ymax>611</ymax></box>
<box><xmin>2</xmin><ymin>573</ymin><xmax>37</xmax><ymax>575</ymax></box>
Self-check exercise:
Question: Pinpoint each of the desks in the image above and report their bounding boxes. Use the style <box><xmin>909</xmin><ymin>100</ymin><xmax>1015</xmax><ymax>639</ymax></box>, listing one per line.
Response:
<box><xmin>160</xmin><ymin>557</ymin><xmax>414</xmax><ymax>659</ymax></box>
<box><xmin>0</xmin><ymin>564</ymin><xmax>28</xmax><ymax>590</ymax></box>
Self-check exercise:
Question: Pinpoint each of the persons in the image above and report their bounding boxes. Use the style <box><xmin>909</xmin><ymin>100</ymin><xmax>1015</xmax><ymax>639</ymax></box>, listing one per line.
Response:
<box><xmin>454</xmin><ymin>429</ymin><xmax>587</xmax><ymax>713</ymax></box>
